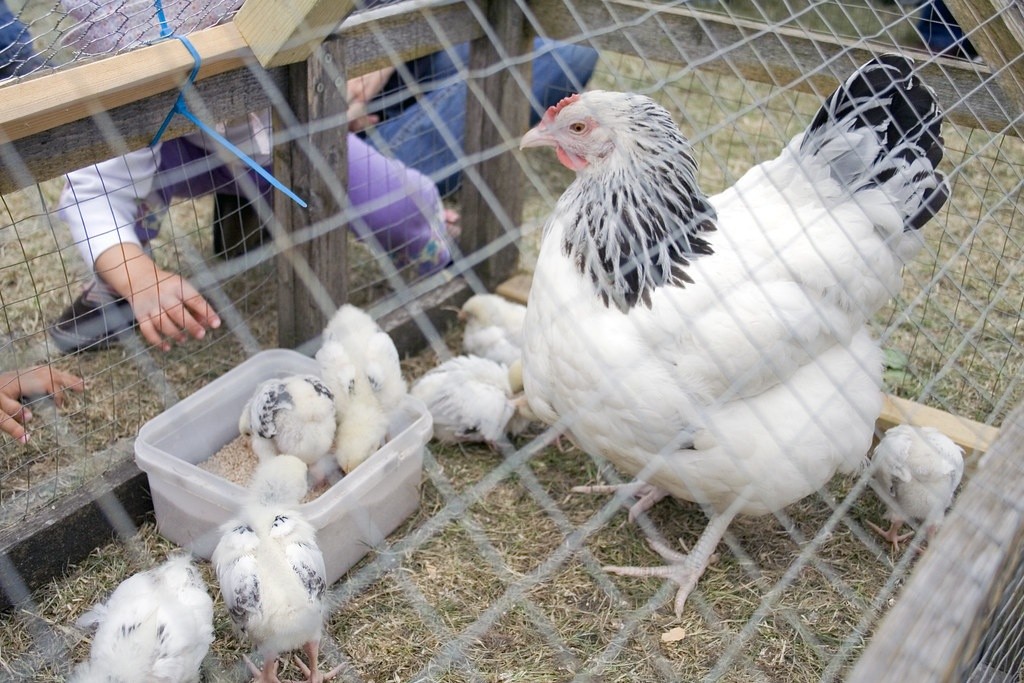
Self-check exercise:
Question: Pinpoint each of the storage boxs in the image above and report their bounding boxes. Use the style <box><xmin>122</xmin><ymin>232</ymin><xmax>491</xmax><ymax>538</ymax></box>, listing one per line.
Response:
<box><xmin>131</xmin><ymin>348</ymin><xmax>433</xmax><ymax>589</ymax></box>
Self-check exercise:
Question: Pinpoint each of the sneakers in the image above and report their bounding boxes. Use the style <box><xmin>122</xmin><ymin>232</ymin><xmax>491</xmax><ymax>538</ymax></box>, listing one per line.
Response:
<box><xmin>214</xmin><ymin>190</ymin><xmax>270</xmax><ymax>259</ymax></box>
<box><xmin>47</xmin><ymin>281</ymin><xmax>138</xmax><ymax>350</ymax></box>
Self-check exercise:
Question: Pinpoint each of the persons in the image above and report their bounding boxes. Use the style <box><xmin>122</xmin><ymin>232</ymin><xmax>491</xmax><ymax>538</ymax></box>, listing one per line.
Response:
<box><xmin>3</xmin><ymin>365</ymin><xmax>90</xmax><ymax>449</ymax></box>
<box><xmin>40</xmin><ymin>4</ymin><xmax>607</xmax><ymax>355</ymax></box>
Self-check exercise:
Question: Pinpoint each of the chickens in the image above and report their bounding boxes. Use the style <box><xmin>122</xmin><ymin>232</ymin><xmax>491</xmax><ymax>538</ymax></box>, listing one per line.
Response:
<box><xmin>238</xmin><ymin>291</ymin><xmax>545</xmax><ymax>503</ymax></box>
<box><xmin>209</xmin><ymin>509</ymin><xmax>347</xmax><ymax>683</ymax></box>
<box><xmin>863</xmin><ymin>423</ymin><xmax>966</xmax><ymax>557</ymax></box>
<box><xmin>520</xmin><ymin>51</ymin><xmax>953</xmax><ymax>621</ymax></box>
<box><xmin>68</xmin><ymin>552</ymin><xmax>217</xmax><ymax>683</ymax></box>
